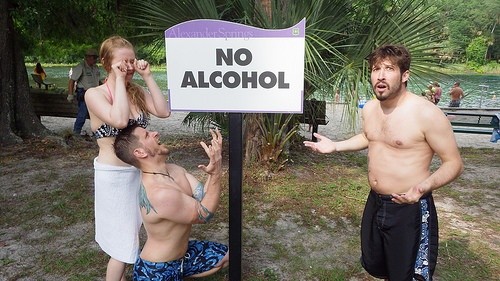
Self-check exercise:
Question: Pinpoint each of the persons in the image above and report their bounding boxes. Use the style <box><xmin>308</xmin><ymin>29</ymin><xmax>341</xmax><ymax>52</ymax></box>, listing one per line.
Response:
<box><xmin>334</xmin><ymin>88</ymin><xmax>342</xmax><ymax>101</ymax></box>
<box><xmin>302</xmin><ymin>45</ymin><xmax>466</xmax><ymax>281</ymax></box>
<box><xmin>418</xmin><ymin>80</ymin><xmax>465</xmax><ymax>107</ymax></box>
<box><xmin>111</xmin><ymin>124</ymin><xmax>229</xmax><ymax>281</ymax></box>
<box><xmin>84</xmin><ymin>35</ymin><xmax>171</xmax><ymax>281</ymax></box>
<box><xmin>66</xmin><ymin>47</ymin><xmax>101</xmax><ymax>134</ymax></box>
<box><xmin>33</xmin><ymin>63</ymin><xmax>47</xmax><ymax>88</ymax></box>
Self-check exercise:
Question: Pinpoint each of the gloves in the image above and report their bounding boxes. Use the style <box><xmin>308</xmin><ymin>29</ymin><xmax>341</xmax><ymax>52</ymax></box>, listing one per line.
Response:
<box><xmin>67</xmin><ymin>94</ymin><xmax>74</xmax><ymax>103</ymax></box>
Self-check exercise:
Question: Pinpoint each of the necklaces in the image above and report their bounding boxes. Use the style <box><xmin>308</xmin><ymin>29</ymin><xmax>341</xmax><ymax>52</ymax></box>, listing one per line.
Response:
<box><xmin>142</xmin><ymin>169</ymin><xmax>175</xmax><ymax>181</ymax></box>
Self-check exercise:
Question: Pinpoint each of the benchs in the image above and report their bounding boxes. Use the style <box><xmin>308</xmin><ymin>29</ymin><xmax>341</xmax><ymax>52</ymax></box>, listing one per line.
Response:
<box><xmin>296</xmin><ymin>98</ymin><xmax>330</xmax><ymax>142</ymax></box>
<box><xmin>30</xmin><ymin>92</ymin><xmax>91</xmax><ymax>119</ymax></box>
<box><xmin>31</xmin><ymin>73</ymin><xmax>55</xmax><ymax>90</ymax></box>
<box><xmin>444</xmin><ymin>112</ymin><xmax>500</xmax><ymax>135</ymax></box>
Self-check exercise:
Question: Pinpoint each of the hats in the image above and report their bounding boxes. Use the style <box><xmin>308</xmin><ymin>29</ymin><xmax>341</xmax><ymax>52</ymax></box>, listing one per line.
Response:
<box><xmin>85</xmin><ymin>49</ymin><xmax>100</xmax><ymax>56</ymax></box>
